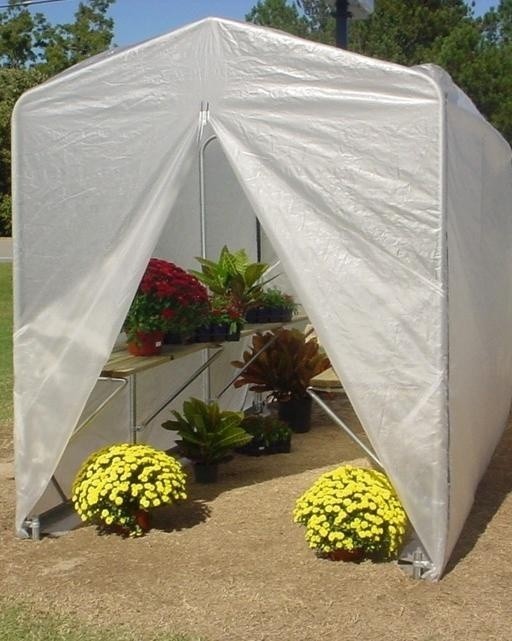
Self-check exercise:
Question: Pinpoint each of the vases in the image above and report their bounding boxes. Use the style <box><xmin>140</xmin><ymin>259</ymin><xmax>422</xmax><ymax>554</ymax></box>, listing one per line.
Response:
<box><xmin>125</xmin><ymin>331</ymin><xmax>164</xmax><ymax>357</ymax></box>
<box><xmin>125</xmin><ymin>509</ymin><xmax>150</xmax><ymax>532</ymax></box>
<box><xmin>333</xmin><ymin>550</ymin><xmax>364</xmax><ymax>564</ymax></box>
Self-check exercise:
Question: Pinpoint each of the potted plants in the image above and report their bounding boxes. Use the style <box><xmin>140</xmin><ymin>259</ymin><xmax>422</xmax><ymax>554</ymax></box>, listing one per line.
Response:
<box><xmin>162</xmin><ymin>326</ymin><xmax>332</xmax><ymax>483</ymax></box>
<box><xmin>189</xmin><ymin>243</ymin><xmax>293</xmax><ymax>341</ymax></box>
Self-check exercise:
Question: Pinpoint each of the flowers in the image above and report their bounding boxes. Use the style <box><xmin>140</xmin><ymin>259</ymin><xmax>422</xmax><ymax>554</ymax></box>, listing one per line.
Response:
<box><xmin>293</xmin><ymin>466</ymin><xmax>406</xmax><ymax>559</ymax></box>
<box><xmin>71</xmin><ymin>441</ymin><xmax>188</xmax><ymax>537</ymax></box>
<box><xmin>120</xmin><ymin>257</ymin><xmax>208</xmax><ymax>347</ymax></box>
